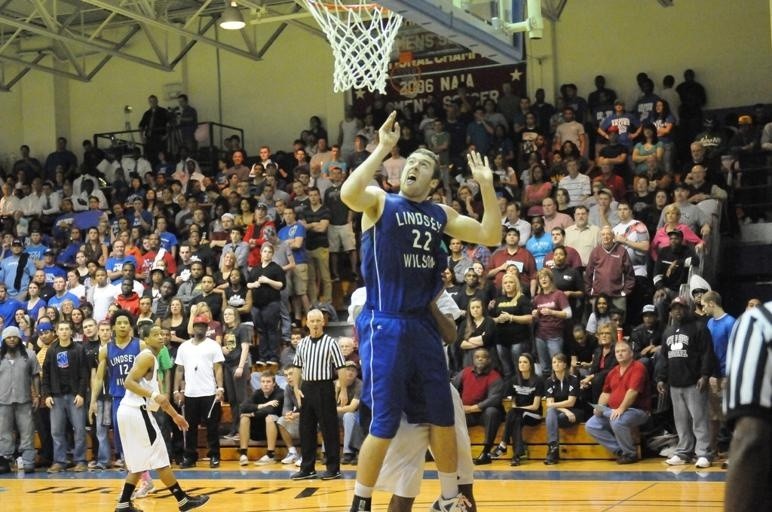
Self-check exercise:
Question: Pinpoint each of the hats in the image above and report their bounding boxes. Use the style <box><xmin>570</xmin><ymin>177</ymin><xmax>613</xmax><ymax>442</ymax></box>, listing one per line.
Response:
<box><xmin>670</xmin><ymin>297</ymin><xmax>689</xmax><ymax>308</ymax></box>
<box><xmin>37</xmin><ymin>322</ymin><xmax>52</xmax><ymax>333</ymax></box>
<box><xmin>192</xmin><ymin>317</ymin><xmax>209</xmax><ymax>327</ymax></box>
<box><xmin>641</xmin><ymin>304</ymin><xmax>659</xmax><ymax>316</ymax></box>
<box><xmin>261</xmin><ymin>226</ymin><xmax>277</xmax><ymax>242</ymax></box>
<box><xmin>598</xmin><ymin>187</ymin><xmax>613</xmax><ymax>199</ymax></box>
<box><xmin>11</xmin><ymin>238</ymin><xmax>25</xmax><ymax>247</ymax></box>
<box><xmin>345</xmin><ymin>360</ymin><xmax>357</xmax><ymax>369</ymax></box>
<box><xmin>43</xmin><ymin>247</ymin><xmax>55</xmax><ymax>256</ymax></box>
<box><xmin>607</xmin><ymin>125</ymin><xmax>619</xmax><ymax>132</ymax></box>
<box><xmin>2</xmin><ymin>326</ymin><xmax>23</xmax><ymax>341</ymax></box>
<box><xmin>666</xmin><ymin>230</ymin><xmax>684</xmax><ymax>239</ymax></box>
<box><xmin>463</xmin><ymin>266</ymin><xmax>479</xmax><ymax>278</ymax></box>
<box><xmin>613</xmin><ymin>98</ymin><xmax>625</xmax><ymax>106</ymax></box>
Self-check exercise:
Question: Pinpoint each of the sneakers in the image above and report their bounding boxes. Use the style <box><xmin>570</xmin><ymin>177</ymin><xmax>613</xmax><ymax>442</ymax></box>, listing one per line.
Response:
<box><xmin>694</xmin><ymin>456</ymin><xmax>712</xmax><ymax>468</ymax></box>
<box><xmin>17</xmin><ymin>465</ymin><xmax>35</xmax><ymax>474</ymax></box>
<box><xmin>90</xmin><ymin>465</ymin><xmax>102</xmax><ymax>471</ymax></box>
<box><xmin>617</xmin><ymin>455</ymin><xmax>639</xmax><ymax>464</ymax></box>
<box><xmin>114</xmin><ymin>502</ymin><xmax>144</xmax><ymax>512</ymax></box>
<box><xmin>118</xmin><ymin>488</ymin><xmax>136</xmax><ymax>502</ymax></box>
<box><xmin>113</xmin><ymin>458</ymin><xmax>125</xmax><ymax>468</ymax></box>
<box><xmin>281</xmin><ymin>452</ymin><xmax>299</xmax><ymax>464</ymax></box>
<box><xmin>74</xmin><ymin>462</ymin><xmax>89</xmax><ymax>472</ymax></box>
<box><xmin>134</xmin><ymin>481</ymin><xmax>156</xmax><ymax>498</ymax></box>
<box><xmin>320</xmin><ymin>470</ymin><xmax>342</xmax><ymax>480</ymax></box>
<box><xmin>46</xmin><ymin>464</ymin><xmax>67</xmax><ymax>474</ymax></box>
<box><xmin>473</xmin><ymin>453</ymin><xmax>493</xmax><ymax>465</ymax></box>
<box><xmin>491</xmin><ymin>447</ymin><xmax>508</xmax><ymax>458</ymax></box>
<box><xmin>253</xmin><ymin>455</ymin><xmax>276</xmax><ymax>467</ymax></box>
<box><xmin>0</xmin><ymin>462</ymin><xmax>12</xmax><ymax>474</ymax></box>
<box><xmin>430</xmin><ymin>491</ymin><xmax>472</xmax><ymax>512</ymax></box>
<box><xmin>238</xmin><ymin>454</ymin><xmax>249</xmax><ymax>466</ymax></box>
<box><xmin>544</xmin><ymin>441</ymin><xmax>560</xmax><ymax>466</ymax></box>
<box><xmin>294</xmin><ymin>457</ymin><xmax>304</xmax><ymax>467</ymax></box>
<box><xmin>178</xmin><ymin>495</ymin><xmax>210</xmax><ymax>512</ymax></box>
<box><xmin>209</xmin><ymin>456</ymin><xmax>221</xmax><ymax>469</ymax></box>
<box><xmin>664</xmin><ymin>455</ymin><xmax>691</xmax><ymax>465</ymax></box>
<box><xmin>511</xmin><ymin>455</ymin><xmax>522</xmax><ymax>467</ymax></box>
<box><xmin>179</xmin><ymin>459</ymin><xmax>196</xmax><ymax>467</ymax></box>
<box><xmin>289</xmin><ymin>469</ymin><xmax>317</xmax><ymax>482</ymax></box>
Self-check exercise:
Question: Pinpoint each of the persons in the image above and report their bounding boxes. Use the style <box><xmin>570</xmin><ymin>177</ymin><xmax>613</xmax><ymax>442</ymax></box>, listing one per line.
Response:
<box><xmin>695</xmin><ymin>114</ymin><xmax>728</xmax><ymax>156</ymax></box>
<box><xmin>632</xmin><ymin>72</ymin><xmax>648</xmax><ymax>113</ymax></box>
<box><xmin>182</xmin><ymin>223</ymin><xmax>210</xmax><ymax>249</ymax></box>
<box><xmin>177</xmin><ymin>206</ymin><xmax>209</xmax><ymax>240</ymax></box>
<box><xmin>451</xmin><ymin>348</ymin><xmax>504</xmax><ymax>468</ymax></box>
<box><xmin>110</xmin><ymin>149</ymin><xmax>136</xmax><ymax>187</ymax></box>
<box><xmin>77</xmin><ymin>302</ymin><xmax>94</xmax><ymax>319</ymax></box>
<box><xmin>130</xmin><ymin>224</ymin><xmax>147</xmax><ymax>255</ymax></box>
<box><xmin>70</xmin><ymin>308</ymin><xmax>86</xmax><ymax>345</ymax></box>
<box><xmin>104</xmin><ymin>239</ymin><xmax>137</xmax><ymax>287</ymax></box>
<box><xmin>661</xmin><ymin>74</ymin><xmax>682</xmax><ymax>126</ymax></box>
<box><xmin>174</xmin><ymin>194</ymin><xmax>190</xmax><ymax>229</ymax></box>
<box><xmin>142</xmin><ymin>267</ymin><xmax>166</xmax><ymax>306</ymax></box>
<box><xmin>29</xmin><ymin>176</ymin><xmax>44</xmax><ymax>200</ymax></box>
<box><xmin>630</xmin><ymin>304</ymin><xmax>664</xmax><ymax>370</ymax></box>
<box><xmin>209</xmin><ymin>212</ymin><xmax>235</xmax><ymax>265</ymax></box>
<box><xmin>492</xmin><ymin>273</ymin><xmax>534</xmax><ymax>397</ymax></box>
<box><xmin>0</xmin><ymin>238</ymin><xmax>38</xmax><ymax>302</ymax></box>
<box><xmin>689</xmin><ymin>274</ymin><xmax>713</xmax><ymax>325</ymax></box>
<box><xmin>644</xmin><ymin>189</ymin><xmax>671</xmax><ymax>242</ymax></box>
<box><xmin>548</xmin><ymin>97</ymin><xmax>566</xmax><ymax>134</ymax></box>
<box><xmin>492</xmin><ymin>150</ymin><xmax>518</xmax><ymax>201</ymax></box>
<box><xmin>189</xmin><ymin>274</ymin><xmax>223</xmax><ymax>321</ymax></box>
<box><xmin>505</xmin><ymin>204</ymin><xmax>531</xmax><ymax>249</ymax></box>
<box><xmin>418</xmin><ymin>104</ymin><xmax>439</xmax><ymax>150</ymax></box>
<box><xmin>497</xmin><ymin>81</ymin><xmax>521</xmax><ymax>126</ymax></box>
<box><xmin>0</xmin><ymin>182</ymin><xmax>20</xmax><ymax>240</ymax></box>
<box><xmin>109</xmin><ymin>168</ymin><xmax>129</xmax><ymax>206</ymax></box>
<box><xmin>0</xmin><ymin>285</ymin><xmax>23</xmax><ymax>327</ymax></box>
<box><xmin>115</xmin><ymin>260</ymin><xmax>145</xmax><ymax>298</ymax></box>
<box><xmin>153</xmin><ymin>201</ymin><xmax>182</xmax><ymax>241</ymax></box>
<box><xmin>729</xmin><ymin>115</ymin><xmax>765</xmax><ymax>223</ymax></box>
<box><xmin>228</xmin><ymin>190</ymin><xmax>242</xmax><ymax>215</ymax></box>
<box><xmin>399</xmin><ymin>105</ymin><xmax>418</xmax><ymax>134</ymax></box>
<box><xmin>534</xmin><ymin>134</ymin><xmax>554</xmax><ymax>178</ymax></box>
<box><xmin>542</xmin><ymin>226</ymin><xmax>583</xmax><ymax>268</ymax></box>
<box><xmin>488</xmin><ymin>228</ymin><xmax>537</xmax><ymax>285</ymax></box>
<box><xmin>580</xmin><ymin>320</ymin><xmax>619</xmax><ymax>404</ymax></box>
<box><xmin>290</xmin><ymin>171</ymin><xmax>312</xmax><ymax>203</ymax></box>
<box><xmin>297</xmin><ymin>186</ymin><xmax>333</xmax><ymax>311</ymax></box>
<box><xmin>66</xmin><ymin>268</ymin><xmax>86</xmax><ymax>299</ymax></box>
<box><xmin>686</xmin><ymin>164</ymin><xmax>728</xmax><ymax>205</ymax></box>
<box><xmin>550</xmin><ymin>140</ymin><xmax>595</xmax><ymax>189</ymax></box>
<box><xmin>240</xmin><ymin>181</ymin><xmax>258</xmax><ymax>213</ymax></box>
<box><xmin>58</xmin><ymin>299</ymin><xmax>75</xmax><ymax>322</ymax></box>
<box><xmin>153</xmin><ymin>216</ymin><xmax>177</xmax><ymax>259</ymax></box>
<box><xmin>260</xmin><ymin>175</ymin><xmax>290</xmax><ymax>205</ymax></box>
<box><xmin>20</xmin><ymin>218</ymin><xmax>51</xmax><ymax>249</ymax></box>
<box><xmin>117</xmin><ymin>228</ymin><xmax>142</xmax><ymax>259</ymax></box>
<box><xmin>348</xmin><ymin>134</ymin><xmax>372</xmax><ymax>175</ymax></box>
<box><xmin>254</xmin><ymin>163</ymin><xmax>288</xmax><ymax>200</ymax></box>
<box><xmin>155</xmin><ymin>149</ymin><xmax>176</xmax><ymax>176</ymax></box>
<box><xmin>554</xmin><ymin>187</ymin><xmax>577</xmax><ymax>222</ymax></box>
<box><xmin>676</xmin><ymin>68</ymin><xmax>707</xmax><ymax>112</ymax></box>
<box><xmin>429</xmin><ymin>119</ymin><xmax>451</xmax><ymax>191</ymax></box>
<box><xmin>88</xmin><ymin>308</ymin><xmax>155</xmax><ymax>503</ymax></box>
<box><xmin>525</xmin><ymin>216</ymin><xmax>555</xmax><ymax>272</ymax></box>
<box><xmin>144</xmin><ymin>188</ymin><xmax>158</xmax><ymax>214</ymax></box>
<box><xmin>585</xmin><ymin>292</ymin><xmax>611</xmax><ymax>338</ymax></box>
<box><xmin>333</xmin><ymin>336</ymin><xmax>362</xmax><ymax>380</ymax></box>
<box><xmin>512</xmin><ymin>96</ymin><xmax>531</xmax><ymax>134</ymax></box>
<box><xmin>86</xmin><ymin>267</ymin><xmax>122</xmax><ymax>325</ymax></box>
<box><xmin>309</xmin><ymin>137</ymin><xmax>343</xmax><ymax>176</ymax></box>
<box><xmin>585</xmin><ymin>340</ymin><xmax>652</xmax><ymax>465</ymax></box>
<box><xmin>108</xmin><ymin>302</ymin><xmax>121</xmax><ymax>320</ymax></box>
<box><xmin>486</xmin><ymin>353</ymin><xmax>544</xmax><ymax>465</ymax></box>
<box><xmin>214</xmin><ymin>157</ymin><xmax>230</xmax><ymax>181</ymax></box>
<box><xmin>450</xmin><ymin>142</ymin><xmax>480</xmax><ymax>198</ymax></box>
<box><xmin>346</xmin><ymin>271</ymin><xmax>477</xmax><ymax>512</ymax></box>
<box><xmin>15</xmin><ymin>168</ymin><xmax>30</xmax><ymax>196</ymax></box>
<box><xmin>62</xmin><ymin>183</ymin><xmax>83</xmax><ymax>211</ymax></box>
<box><xmin>206</xmin><ymin>182</ymin><xmax>230</xmax><ymax>220</ymax></box>
<box><xmin>217</xmin><ymin>176</ymin><xmax>226</xmax><ymax>191</ymax></box>
<box><xmin>454</xmin><ymin>81</ymin><xmax>476</xmax><ymax>125</ymax></box>
<box><xmin>337</xmin><ymin>104</ymin><xmax>364</xmax><ymax>161</ymax></box>
<box><xmin>209</xmin><ymin>203</ymin><xmax>226</xmax><ymax>232</ymax></box>
<box><xmin>234</xmin><ymin>197</ymin><xmax>256</xmax><ymax>235</ymax></box>
<box><xmin>530</xmin><ymin>88</ymin><xmax>555</xmax><ymax>151</ymax></box>
<box><xmin>498</xmin><ymin>263</ymin><xmax>530</xmax><ymax>297</ymax></box>
<box><xmin>57</xmin><ymin>176</ymin><xmax>73</xmax><ymax>210</ymax></box>
<box><xmin>309</xmin><ymin>115</ymin><xmax>328</xmax><ymax>144</ymax></box>
<box><xmin>699</xmin><ymin>290</ymin><xmax>737</xmax><ymax>470</ymax></box>
<box><xmin>138</xmin><ymin>94</ymin><xmax>172</xmax><ymax>172</ymax></box>
<box><xmin>77</xmin><ymin>179</ymin><xmax>109</xmax><ymax>210</ymax></box>
<box><xmin>118</xmin><ymin>216</ymin><xmax>131</xmax><ymax>233</ymax></box>
<box><xmin>276</xmin><ymin>363</ymin><xmax>303</xmax><ymax>467</ymax></box>
<box><xmin>80</xmin><ymin>226</ymin><xmax>109</xmax><ymax>269</ymax></box>
<box><xmin>178</xmin><ymin>195</ymin><xmax>210</xmax><ymax>240</ymax></box>
<box><xmin>145</xmin><ymin>170</ymin><xmax>158</xmax><ymax>192</ymax></box>
<box><xmin>175</xmin><ymin>255</ymin><xmax>207</xmax><ymax>316</ymax></box>
<box><xmin>187</xmin><ymin>301</ymin><xmax>222</xmax><ymax>345</ymax></box>
<box><xmin>161</xmin><ymin>327</ymin><xmax>184</xmax><ymax>465</ymax></box>
<box><xmin>483</xmin><ymin>98</ymin><xmax>510</xmax><ymax>133</ymax></box>
<box><xmin>30</xmin><ymin>322</ymin><xmax>58</xmax><ymax>468</ymax></box>
<box><xmin>682</xmin><ymin>140</ymin><xmax>720</xmax><ymax>184</ymax></box>
<box><xmin>397</xmin><ymin>122</ymin><xmax>418</xmax><ymax>159</ymax></box>
<box><xmin>25</xmin><ymin>269</ymin><xmax>56</xmax><ymax>306</ymax></box>
<box><xmin>14</xmin><ymin>307</ymin><xmax>28</xmax><ymax>323</ymax></box>
<box><xmin>304</xmin><ymin>133</ymin><xmax>319</xmax><ymax>157</ymax></box>
<box><xmin>380</xmin><ymin>144</ymin><xmax>407</xmax><ymax>194</ymax></box>
<box><xmin>456</xmin><ymin>267</ymin><xmax>489</xmax><ymax>315</ymax></box>
<box><xmin>183</xmin><ymin>159</ymin><xmax>207</xmax><ymax>194</ymax></box>
<box><xmin>123</xmin><ymin>175</ymin><xmax>147</xmax><ymax>214</ymax></box>
<box><xmin>246</xmin><ymin>241</ymin><xmax>287</xmax><ymax>367</ymax></box>
<box><xmin>132</xmin><ymin>146</ymin><xmax>152</xmax><ymax>185</ymax></box>
<box><xmin>38</xmin><ymin>247</ymin><xmax>66</xmax><ymax>288</ymax></box>
<box><xmin>42</xmin><ymin>321</ymin><xmax>88</xmax><ymax>472</ymax></box>
<box><xmin>128</xmin><ymin>196</ymin><xmax>153</xmax><ymax>235</ymax></box>
<box><xmin>188</xmin><ymin>230</ymin><xmax>214</xmax><ymax>276</ymax></box>
<box><xmin>564</xmin><ymin>84</ymin><xmax>590</xmax><ymax>158</ymax></box>
<box><xmin>543</xmin><ymin>352</ymin><xmax>584</xmax><ymax>465</ymax></box>
<box><xmin>457</xmin><ymin>185</ymin><xmax>484</xmax><ymax>223</ymax></box>
<box><xmin>760</xmin><ymin>122</ymin><xmax>772</xmax><ymax>185</ymax></box>
<box><xmin>0</xmin><ymin>232</ymin><xmax>15</xmax><ymax>261</ymax></box>
<box><xmin>585</xmin><ymin>225</ymin><xmax>635</xmax><ymax>318</ymax></box>
<box><xmin>466</xmin><ymin>106</ymin><xmax>495</xmax><ymax>157</ymax></box>
<box><xmin>751</xmin><ymin>103</ymin><xmax>767</xmax><ymax>128</ymax></box>
<box><xmin>82</xmin><ymin>138</ymin><xmax>106</xmax><ymax>176</ymax></box>
<box><xmin>589</xmin><ymin>188</ymin><xmax>622</xmax><ymax>228</ymax></box>
<box><xmin>172</xmin><ymin>316</ymin><xmax>225</xmax><ymax>468</ymax></box>
<box><xmin>357</xmin><ymin>112</ymin><xmax>376</xmax><ymax>144</ymax></box>
<box><xmin>171</xmin><ymin>180</ymin><xmax>183</xmax><ymax>204</ymax></box>
<box><xmin>151</xmin><ymin>277</ymin><xmax>178</xmax><ymax>321</ymax></box>
<box><xmin>134</xmin><ymin>232</ymin><xmax>177</xmax><ymax>290</ymax></box>
<box><xmin>175</xmin><ymin>146</ymin><xmax>201</xmax><ymax>176</ymax></box>
<box><xmin>221</xmin><ymin>173</ymin><xmax>244</xmax><ymax>201</ymax></box>
<box><xmin>222</xmin><ymin>304</ymin><xmax>252</xmax><ymax>441</ymax></box>
<box><xmin>455</xmin><ymin>296</ymin><xmax>501</xmax><ymax>370</ymax></box>
<box><xmin>161</xmin><ymin>298</ymin><xmax>190</xmax><ymax>349</ymax></box>
<box><xmin>339</xmin><ymin>110</ymin><xmax>503</xmax><ymax>512</ymax></box>
<box><xmin>633</xmin><ymin>78</ymin><xmax>660</xmax><ymax>124</ymax></box>
<box><xmin>443</xmin><ymin>265</ymin><xmax>461</xmax><ymax>301</ymax></box>
<box><xmin>462</xmin><ymin>241</ymin><xmax>492</xmax><ymax>269</ymax></box>
<box><xmin>171</xmin><ymin>94</ymin><xmax>199</xmax><ymax>160</ymax></box>
<box><xmin>82</xmin><ymin>318</ymin><xmax>101</xmax><ymax>468</ymax></box>
<box><xmin>22</xmin><ymin>281</ymin><xmax>46</xmax><ymax>336</ymax></box>
<box><xmin>113</xmin><ymin>324</ymin><xmax>209</xmax><ymax>512</ymax></box>
<box><xmin>446</xmin><ymin>237</ymin><xmax>472</xmax><ymax>275</ymax></box>
<box><xmin>250</xmin><ymin>163</ymin><xmax>266</xmax><ymax>211</ymax></box>
<box><xmin>309</xmin><ymin>163</ymin><xmax>333</xmax><ymax>205</ymax></box>
<box><xmin>226</xmin><ymin>151</ymin><xmax>250</xmax><ymax>183</ymax></box>
<box><xmin>46</xmin><ymin>305</ymin><xmax>60</xmax><ymax>335</ymax></box>
<box><xmin>6</xmin><ymin>173</ymin><xmax>23</xmax><ymax>199</ymax></box>
<box><xmin>83</xmin><ymin>260</ymin><xmax>111</xmax><ymax>296</ymax></box>
<box><xmin>257</xmin><ymin>184</ymin><xmax>278</xmax><ymax>223</ymax></box>
<box><xmin>626</xmin><ymin>174</ymin><xmax>654</xmax><ymax>221</ymax></box>
<box><xmin>287</xmin><ymin>180</ymin><xmax>309</xmax><ymax>222</ymax></box>
<box><xmin>213</xmin><ymin>268</ymin><xmax>254</xmax><ymax>326</ymax></box>
<box><xmin>174</xmin><ymin>243</ymin><xmax>192</xmax><ymax>291</ymax></box>
<box><xmin>278</xmin><ymin>140</ymin><xmax>312</xmax><ymax>191</ymax></box>
<box><xmin>276</xmin><ymin>206</ymin><xmax>310</xmax><ymax>328</ymax></box>
<box><xmin>551</xmin><ymin>245</ymin><xmax>585</xmax><ymax>356</ymax></box>
<box><xmin>596</xmin><ymin>98</ymin><xmax>640</xmax><ymax>172</ymax></box>
<box><xmin>652</xmin><ymin>228</ymin><xmax>701</xmax><ymax>303</ymax></box>
<box><xmin>653</xmin><ymin>98</ymin><xmax>676</xmax><ymax>175</ymax></box>
<box><xmin>259</xmin><ymin>226</ymin><xmax>296</xmax><ymax>345</ymax></box>
<box><xmin>0</xmin><ymin>326</ymin><xmax>42</xmax><ymax>475</ymax></box>
<box><xmin>115</xmin><ymin>277</ymin><xmax>142</xmax><ymax>317</ymax></box>
<box><xmin>632</xmin><ymin>121</ymin><xmax>664</xmax><ymax>192</ymax></box>
<box><xmin>104</xmin><ymin>201</ymin><xmax>131</xmax><ymax>244</ymax></box>
<box><xmin>249</xmin><ymin>330</ymin><xmax>305</xmax><ymax>392</ymax></box>
<box><xmin>651</xmin><ymin>206</ymin><xmax>706</xmax><ymax>262</ymax></box>
<box><xmin>587</xmin><ymin>75</ymin><xmax>617</xmax><ymax>114</ymax></box>
<box><xmin>19</xmin><ymin>314</ymin><xmax>35</xmax><ymax>347</ymax></box>
<box><xmin>12</xmin><ymin>145</ymin><xmax>42</xmax><ymax>184</ymax></box>
<box><xmin>519</xmin><ymin>151</ymin><xmax>542</xmax><ymax>188</ymax></box>
<box><xmin>55</xmin><ymin>226</ymin><xmax>85</xmax><ymax>273</ymax></box>
<box><xmin>132</xmin><ymin>295</ymin><xmax>161</xmax><ymax>338</ymax></box>
<box><xmin>218</xmin><ymin>226</ymin><xmax>250</xmax><ymax>281</ymax></box>
<box><xmin>722</xmin><ymin>300</ymin><xmax>772</xmax><ymax>512</ymax></box>
<box><xmin>52</xmin><ymin>171</ymin><xmax>65</xmax><ymax>191</ymax></box>
<box><xmin>156</xmin><ymin>173</ymin><xmax>171</xmax><ymax>203</ymax></box>
<box><xmin>73</xmin><ymin>163</ymin><xmax>99</xmax><ymax>194</ymax></box>
<box><xmin>243</xmin><ymin>204</ymin><xmax>274</xmax><ymax>272</ymax></box>
<box><xmin>657</xmin><ymin>183</ymin><xmax>711</xmax><ymax>237</ymax></box>
<box><xmin>213</xmin><ymin>252</ymin><xmax>238</xmax><ymax>286</ymax></box>
<box><xmin>606</xmin><ymin>308</ymin><xmax>625</xmax><ymax>328</ymax></box>
<box><xmin>451</xmin><ymin>199</ymin><xmax>468</xmax><ymax>216</ymax></box>
<box><xmin>239</xmin><ymin>371</ymin><xmax>285</xmax><ymax>466</ymax></box>
<box><xmin>161</xmin><ymin>186</ymin><xmax>184</xmax><ymax>223</ymax></box>
<box><xmin>654</xmin><ymin>296</ymin><xmax>714</xmax><ymax>469</ymax></box>
<box><xmin>427</xmin><ymin>192</ymin><xmax>443</xmax><ymax>203</ymax></box>
<box><xmin>23</xmin><ymin>229</ymin><xmax>47</xmax><ymax>270</ymax></box>
<box><xmin>530</xmin><ymin>268</ymin><xmax>573</xmax><ymax>372</ymax></box>
<box><xmin>444</xmin><ymin>105</ymin><xmax>466</xmax><ymax>162</ymax></box>
<box><xmin>597</xmin><ymin>125</ymin><xmax>628</xmax><ymax>178</ymax></box>
<box><xmin>516</xmin><ymin>111</ymin><xmax>541</xmax><ymax>170</ymax></box>
<box><xmin>564</xmin><ymin>205</ymin><xmax>601</xmax><ymax>267</ymax></box>
<box><xmin>140</xmin><ymin>234</ymin><xmax>152</xmax><ymax>255</ymax></box>
<box><xmin>90</xmin><ymin>320</ymin><xmax>112</xmax><ymax>471</ymax></box>
<box><xmin>40</xmin><ymin>180</ymin><xmax>63</xmax><ymax>227</ymax></box>
<box><xmin>320</xmin><ymin>361</ymin><xmax>363</xmax><ymax>464</ymax></box>
<box><xmin>593</xmin><ymin>89</ymin><xmax>616</xmax><ymax>162</ymax></box>
<box><xmin>323</xmin><ymin>167</ymin><xmax>359</xmax><ymax>282</ymax></box>
<box><xmin>48</xmin><ymin>274</ymin><xmax>82</xmax><ymax>311</ymax></box>
<box><xmin>248</xmin><ymin>146</ymin><xmax>278</xmax><ymax>179</ymax></box>
<box><xmin>85</xmin><ymin>195</ymin><xmax>109</xmax><ymax>244</ymax></box>
<box><xmin>612</xmin><ymin>199</ymin><xmax>650</xmax><ymax>324</ymax></box>
<box><xmin>323</xmin><ymin>143</ymin><xmax>348</xmax><ymax>188</ymax></box>
<box><xmin>570</xmin><ymin>322</ymin><xmax>599</xmax><ymax>363</ymax></box>
<box><xmin>17</xmin><ymin>181</ymin><xmax>40</xmax><ymax>236</ymax></box>
<box><xmin>74</xmin><ymin>250</ymin><xmax>92</xmax><ymax>285</ymax></box>
<box><xmin>273</xmin><ymin>198</ymin><xmax>287</xmax><ymax>233</ymax></box>
<box><xmin>541</xmin><ymin>196</ymin><xmax>573</xmax><ymax>233</ymax></box>
<box><xmin>184</xmin><ymin>178</ymin><xmax>208</xmax><ymax>204</ymax></box>
<box><xmin>554</xmin><ymin>106</ymin><xmax>586</xmax><ymax>163</ymax></box>
<box><xmin>591</xmin><ymin>159</ymin><xmax>627</xmax><ymax>203</ymax></box>
<box><xmin>292</xmin><ymin>149</ymin><xmax>310</xmax><ymax>181</ymax></box>
<box><xmin>28</xmin><ymin>316</ymin><xmax>52</xmax><ymax>356</ymax></box>
<box><xmin>0</xmin><ymin>315</ymin><xmax>6</xmax><ymax>348</ymax></box>
<box><xmin>521</xmin><ymin>164</ymin><xmax>553</xmax><ymax>222</ymax></box>
<box><xmin>51</xmin><ymin>198</ymin><xmax>76</xmax><ymax>248</ymax></box>
<box><xmin>472</xmin><ymin>260</ymin><xmax>499</xmax><ymax>314</ymax></box>
<box><xmin>225</xmin><ymin>134</ymin><xmax>249</xmax><ymax>167</ymax></box>
<box><xmin>558</xmin><ymin>159</ymin><xmax>592</xmax><ymax>207</ymax></box>
<box><xmin>137</xmin><ymin>318</ymin><xmax>172</xmax><ymax>463</ymax></box>
<box><xmin>495</xmin><ymin>122</ymin><xmax>515</xmax><ymax>161</ymax></box>
<box><xmin>44</xmin><ymin>135</ymin><xmax>74</xmax><ymax>188</ymax></box>
